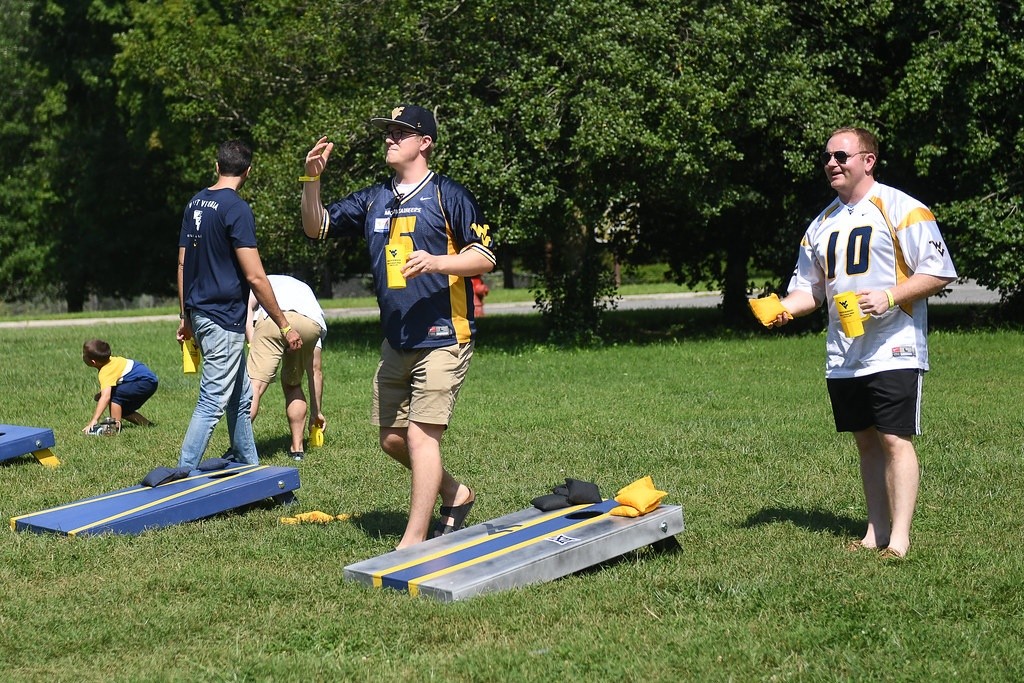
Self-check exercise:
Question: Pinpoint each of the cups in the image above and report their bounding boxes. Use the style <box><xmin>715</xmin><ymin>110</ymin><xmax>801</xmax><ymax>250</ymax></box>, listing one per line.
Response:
<box><xmin>385</xmin><ymin>244</ymin><xmax>415</xmax><ymax>290</ymax></box>
<box><xmin>834</xmin><ymin>291</ymin><xmax>870</xmax><ymax>339</ymax></box>
<box><xmin>181</xmin><ymin>339</ymin><xmax>200</xmax><ymax>374</ymax></box>
<box><xmin>309</xmin><ymin>427</ymin><xmax>325</xmax><ymax>447</ymax></box>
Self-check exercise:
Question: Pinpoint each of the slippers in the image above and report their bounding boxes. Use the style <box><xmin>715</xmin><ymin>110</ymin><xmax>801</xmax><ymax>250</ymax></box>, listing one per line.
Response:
<box><xmin>433</xmin><ymin>487</ymin><xmax>476</xmax><ymax>537</ymax></box>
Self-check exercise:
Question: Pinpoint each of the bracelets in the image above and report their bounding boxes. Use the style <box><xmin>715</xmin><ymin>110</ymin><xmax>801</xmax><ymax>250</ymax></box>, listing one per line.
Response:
<box><xmin>298</xmin><ymin>173</ymin><xmax>320</xmax><ymax>181</ymax></box>
<box><xmin>279</xmin><ymin>324</ymin><xmax>292</xmax><ymax>335</ymax></box>
<box><xmin>180</xmin><ymin>312</ymin><xmax>187</xmax><ymax>319</ymax></box>
<box><xmin>884</xmin><ymin>288</ymin><xmax>895</xmax><ymax>312</ymax></box>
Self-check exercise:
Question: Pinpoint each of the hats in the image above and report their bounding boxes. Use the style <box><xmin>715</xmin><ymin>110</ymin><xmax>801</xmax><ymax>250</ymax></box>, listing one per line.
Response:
<box><xmin>371</xmin><ymin>104</ymin><xmax>437</xmax><ymax>143</ymax></box>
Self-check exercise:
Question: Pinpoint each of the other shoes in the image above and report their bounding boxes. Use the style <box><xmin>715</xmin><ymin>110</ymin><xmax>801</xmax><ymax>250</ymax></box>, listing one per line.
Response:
<box><xmin>86</xmin><ymin>423</ymin><xmax>121</xmax><ymax>436</ymax></box>
<box><xmin>283</xmin><ymin>439</ymin><xmax>304</xmax><ymax>461</ymax></box>
<box><xmin>879</xmin><ymin>544</ymin><xmax>911</xmax><ymax>563</ymax></box>
<box><xmin>848</xmin><ymin>539</ymin><xmax>889</xmax><ymax>553</ymax></box>
<box><xmin>222</xmin><ymin>444</ymin><xmax>258</xmax><ymax>460</ymax></box>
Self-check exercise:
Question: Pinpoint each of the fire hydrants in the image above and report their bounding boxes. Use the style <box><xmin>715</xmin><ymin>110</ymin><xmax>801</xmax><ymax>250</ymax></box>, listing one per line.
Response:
<box><xmin>469</xmin><ymin>274</ymin><xmax>490</xmax><ymax>318</ymax></box>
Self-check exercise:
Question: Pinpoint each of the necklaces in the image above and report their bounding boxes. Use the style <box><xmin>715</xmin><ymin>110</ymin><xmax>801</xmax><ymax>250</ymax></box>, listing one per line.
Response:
<box><xmin>845</xmin><ymin>194</ymin><xmax>867</xmax><ymax>215</ymax></box>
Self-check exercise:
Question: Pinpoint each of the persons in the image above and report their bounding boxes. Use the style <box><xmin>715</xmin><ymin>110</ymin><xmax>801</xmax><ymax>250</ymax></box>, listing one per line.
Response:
<box><xmin>244</xmin><ymin>274</ymin><xmax>328</xmax><ymax>461</ymax></box>
<box><xmin>177</xmin><ymin>139</ymin><xmax>303</xmax><ymax>470</ymax></box>
<box><xmin>299</xmin><ymin>103</ymin><xmax>498</xmax><ymax>554</ymax></box>
<box><xmin>82</xmin><ymin>337</ymin><xmax>159</xmax><ymax>437</ymax></box>
<box><xmin>757</xmin><ymin>124</ymin><xmax>957</xmax><ymax>561</ymax></box>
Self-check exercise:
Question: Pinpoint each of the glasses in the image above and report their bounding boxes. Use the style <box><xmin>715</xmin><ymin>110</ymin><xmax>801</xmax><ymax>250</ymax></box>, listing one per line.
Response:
<box><xmin>380</xmin><ymin>129</ymin><xmax>427</xmax><ymax>141</ymax></box>
<box><xmin>820</xmin><ymin>150</ymin><xmax>878</xmax><ymax>164</ymax></box>
